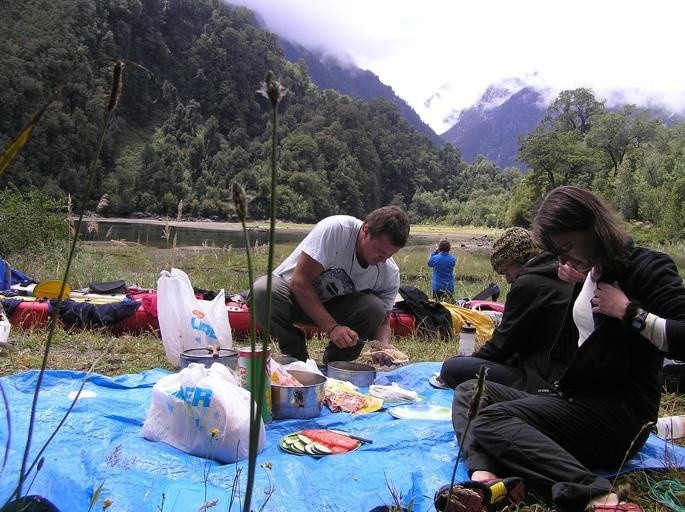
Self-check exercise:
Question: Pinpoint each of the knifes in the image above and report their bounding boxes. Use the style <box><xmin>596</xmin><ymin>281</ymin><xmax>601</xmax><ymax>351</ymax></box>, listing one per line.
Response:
<box><xmin>330</xmin><ymin>429</ymin><xmax>373</xmax><ymax>445</ymax></box>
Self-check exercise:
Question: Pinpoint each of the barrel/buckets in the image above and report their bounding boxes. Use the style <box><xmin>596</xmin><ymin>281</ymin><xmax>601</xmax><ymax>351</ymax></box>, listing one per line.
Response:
<box><xmin>179</xmin><ymin>346</ymin><xmax>241</xmax><ymax>387</ymax></box>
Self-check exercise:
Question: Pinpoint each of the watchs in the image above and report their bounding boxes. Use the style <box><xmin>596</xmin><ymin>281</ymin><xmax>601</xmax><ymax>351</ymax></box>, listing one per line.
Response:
<box><xmin>631</xmin><ymin>312</ymin><xmax>648</xmax><ymax>331</ymax></box>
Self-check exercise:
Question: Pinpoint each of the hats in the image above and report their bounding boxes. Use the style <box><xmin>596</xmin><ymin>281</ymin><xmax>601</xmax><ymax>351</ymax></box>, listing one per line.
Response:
<box><xmin>490</xmin><ymin>226</ymin><xmax>539</xmax><ymax>273</ymax></box>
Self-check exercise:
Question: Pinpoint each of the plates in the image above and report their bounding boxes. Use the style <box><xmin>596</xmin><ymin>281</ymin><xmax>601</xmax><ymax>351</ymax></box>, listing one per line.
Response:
<box><xmin>429</xmin><ymin>376</ymin><xmax>449</xmax><ymax>389</ymax></box>
<box><xmin>388</xmin><ymin>403</ymin><xmax>452</xmax><ymax>422</ymax></box>
<box><xmin>279</xmin><ymin>430</ymin><xmax>362</xmax><ymax>458</ymax></box>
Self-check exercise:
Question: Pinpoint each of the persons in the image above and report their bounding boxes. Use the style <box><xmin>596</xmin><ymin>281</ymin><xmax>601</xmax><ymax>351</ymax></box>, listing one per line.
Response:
<box><xmin>428</xmin><ymin>240</ymin><xmax>456</xmax><ymax>303</ymax></box>
<box><xmin>437</xmin><ymin>227</ymin><xmax>572</xmax><ymax>394</ymax></box>
<box><xmin>435</xmin><ymin>186</ymin><xmax>684</xmax><ymax>512</ymax></box>
<box><xmin>246</xmin><ymin>205</ymin><xmax>410</xmax><ymax>365</ymax></box>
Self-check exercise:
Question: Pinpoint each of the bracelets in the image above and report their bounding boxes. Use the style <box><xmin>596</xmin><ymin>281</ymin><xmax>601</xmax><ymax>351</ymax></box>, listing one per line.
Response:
<box><xmin>328</xmin><ymin>324</ymin><xmax>342</xmax><ymax>339</ymax></box>
<box><xmin>623</xmin><ymin>301</ymin><xmax>642</xmax><ymax>322</ymax></box>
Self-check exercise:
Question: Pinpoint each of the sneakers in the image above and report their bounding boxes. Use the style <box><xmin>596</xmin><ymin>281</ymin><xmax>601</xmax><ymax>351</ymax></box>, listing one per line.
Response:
<box><xmin>582</xmin><ymin>502</ymin><xmax>642</xmax><ymax>512</ymax></box>
<box><xmin>434</xmin><ymin>477</ymin><xmax>526</xmax><ymax>512</ymax></box>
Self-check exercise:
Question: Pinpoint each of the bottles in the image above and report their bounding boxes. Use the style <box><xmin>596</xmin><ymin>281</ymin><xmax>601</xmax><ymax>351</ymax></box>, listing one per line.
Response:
<box><xmin>458</xmin><ymin>321</ymin><xmax>477</xmax><ymax>358</ymax></box>
<box><xmin>653</xmin><ymin>415</ymin><xmax>685</xmax><ymax>440</ymax></box>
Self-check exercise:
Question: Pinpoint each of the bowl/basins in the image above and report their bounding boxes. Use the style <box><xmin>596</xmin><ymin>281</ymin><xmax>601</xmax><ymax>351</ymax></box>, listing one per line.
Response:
<box><xmin>328</xmin><ymin>361</ymin><xmax>377</xmax><ymax>387</ymax></box>
